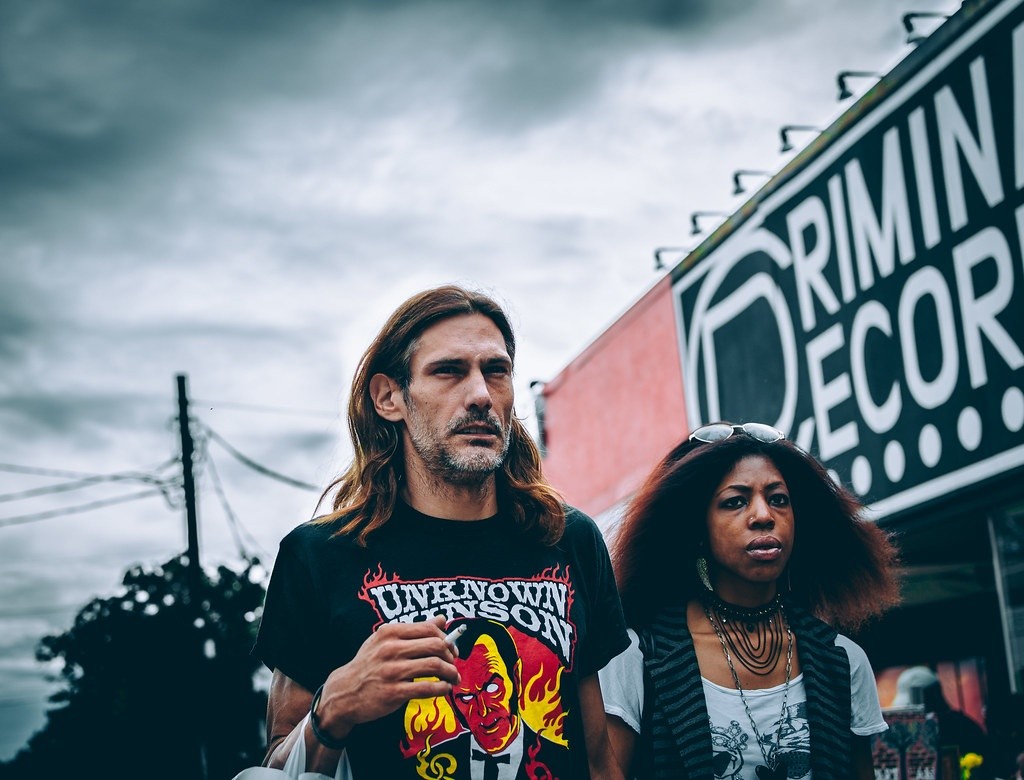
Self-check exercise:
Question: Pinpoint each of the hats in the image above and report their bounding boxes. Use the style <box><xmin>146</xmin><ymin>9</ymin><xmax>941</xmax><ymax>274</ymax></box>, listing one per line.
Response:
<box><xmin>890</xmin><ymin>666</ymin><xmax>938</xmax><ymax>707</ymax></box>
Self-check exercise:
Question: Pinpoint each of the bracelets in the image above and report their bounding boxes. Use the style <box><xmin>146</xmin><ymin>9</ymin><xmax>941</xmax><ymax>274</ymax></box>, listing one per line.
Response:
<box><xmin>310</xmin><ymin>684</ymin><xmax>357</xmax><ymax>752</ymax></box>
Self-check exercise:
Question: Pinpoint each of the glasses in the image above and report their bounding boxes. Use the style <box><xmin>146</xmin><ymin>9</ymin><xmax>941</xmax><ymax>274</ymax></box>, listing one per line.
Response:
<box><xmin>688</xmin><ymin>422</ymin><xmax>784</xmax><ymax>443</ymax></box>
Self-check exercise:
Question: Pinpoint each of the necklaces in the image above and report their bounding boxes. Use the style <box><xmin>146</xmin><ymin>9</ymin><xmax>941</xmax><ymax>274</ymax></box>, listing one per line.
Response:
<box><xmin>703</xmin><ymin>593</ymin><xmax>794</xmax><ymax>780</ymax></box>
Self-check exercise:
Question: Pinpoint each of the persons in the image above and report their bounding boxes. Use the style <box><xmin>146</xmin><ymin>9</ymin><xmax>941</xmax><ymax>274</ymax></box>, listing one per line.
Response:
<box><xmin>251</xmin><ymin>287</ymin><xmax>632</xmax><ymax>780</ymax></box>
<box><xmin>598</xmin><ymin>419</ymin><xmax>889</xmax><ymax>780</ymax></box>
